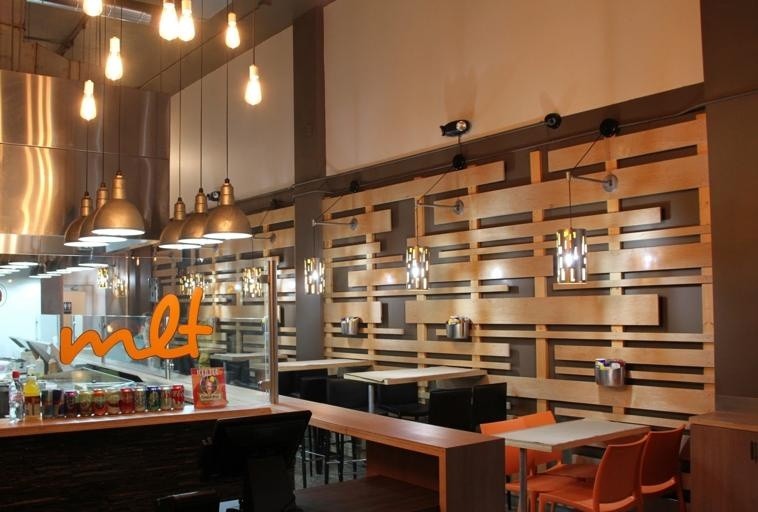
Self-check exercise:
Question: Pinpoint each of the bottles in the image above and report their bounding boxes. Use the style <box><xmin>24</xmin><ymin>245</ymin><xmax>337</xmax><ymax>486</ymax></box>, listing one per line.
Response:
<box><xmin>7</xmin><ymin>370</ymin><xmax>42</xmax><ymax>424</ymax></box>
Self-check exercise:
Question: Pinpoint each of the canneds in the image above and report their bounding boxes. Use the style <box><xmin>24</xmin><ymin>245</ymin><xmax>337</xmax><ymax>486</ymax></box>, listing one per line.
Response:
<box><xmin>63</xmin><ymin>391</ymin><xmax>79</xmax><ymax>417</ymax></box>
<box><xmin>105</xmin><ymin>388</ymin><xmax>120</xmax><ymax>415</ymax></box>
<box><xmin>92</xmin><ymin>389</ymin><xmax>106</xmax><ymax>416</ymax></box>
<box><xmin>79</xmin><ymin>390</ymin><xmax>92</xmax><ymax>417</ymax></box>
<box><xmin>171</xmin><ymin>384</ymin><xmax>184</xmax><ymax>411</ymax></box>
<box><xmin>119</xmin><ymin>388</ymin><xmax>134</xmax><ymax>414</ymax></box>
<box><xmin>146</xmin><ymin>386</ymin><xmax>161</xmax><ymax>412</ymax></box>
<box><xmin>159</xmin><ymin>385</ymin><xmax>172</xmax><ymax>411</ymax></box>
<box><xmin>41</xmin><ymin>389</ymin><xmax>52</xmax><ymax>417</ymax></box>
<box><xmin>134</xmin><ymin>388</ymin><xmax>147</xmax><ymax>412</ymax></box>
<box><xmin>51</xmin><ymin>389</ymin><xmax>65</xmax><ymax>417</ymax></box>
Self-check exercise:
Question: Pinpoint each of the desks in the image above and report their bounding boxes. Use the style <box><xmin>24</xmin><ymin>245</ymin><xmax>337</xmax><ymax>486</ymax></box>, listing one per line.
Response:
<box><xmin>687</xmin><ymin>409</ymin><xmax>757</xmax><ymax>512</ymax></box>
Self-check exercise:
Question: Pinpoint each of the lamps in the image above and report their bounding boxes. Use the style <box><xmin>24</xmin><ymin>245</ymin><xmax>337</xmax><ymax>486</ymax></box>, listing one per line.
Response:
<box><xmin>555</xmin><ymin>116</ymin><xmax>618</xmax><ymax>285</ymax></box>
<box><xmin>0</xmin><ymin>253</ymin><xmax>141</xmax><ymax>298</ymax></box>
<box><xmin>303</xmin><ymin>179</ymin><xmax>360</xmax><ymax>295</ymax></box>
<box><xmin>405</xmin><ymin>153</ymin><xmax>468</xmax><ymax>291</ymax></box>
<box><xmin>62</xmin><ymin>0</ymin><xmax>146</xmax><ymax>250</ymax></box>
<box><xmin>174</xmin><ymin>196</ymin><xmax>280</xmax><ymax>300</ymax></box>
<box><xmin>157</xmin><ymin>0</ymin><xmax>263</xmax><ymax>252</ymax></box>
